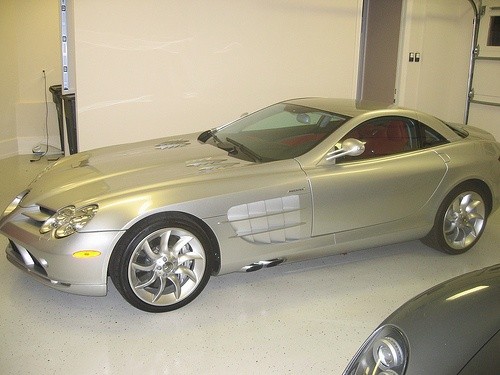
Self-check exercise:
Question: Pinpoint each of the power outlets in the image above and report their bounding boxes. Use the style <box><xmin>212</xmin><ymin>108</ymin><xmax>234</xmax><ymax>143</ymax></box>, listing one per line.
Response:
<box><xmin>41</xmin><ymin>70</ymin><xmax>47</xmax><ymax>78</ymax></box>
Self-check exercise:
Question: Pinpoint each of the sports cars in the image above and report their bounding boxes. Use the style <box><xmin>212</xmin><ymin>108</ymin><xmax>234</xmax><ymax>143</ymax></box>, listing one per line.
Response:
<box><xmin>1</xmin><ymin>95</ymin><xmax>499</xmax><ymax>313</ymax></box>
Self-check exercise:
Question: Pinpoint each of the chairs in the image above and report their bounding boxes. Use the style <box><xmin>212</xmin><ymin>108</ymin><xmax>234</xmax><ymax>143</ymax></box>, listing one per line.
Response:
<box><xmin>276</xmin><ymin>132</ymin><xmax>325</xmax><ymax>146</ymax></box>
<box><xmin>352</xmin><ymin>122</ymin><xmax>407</xmax><ymax>160</ymax></box>
